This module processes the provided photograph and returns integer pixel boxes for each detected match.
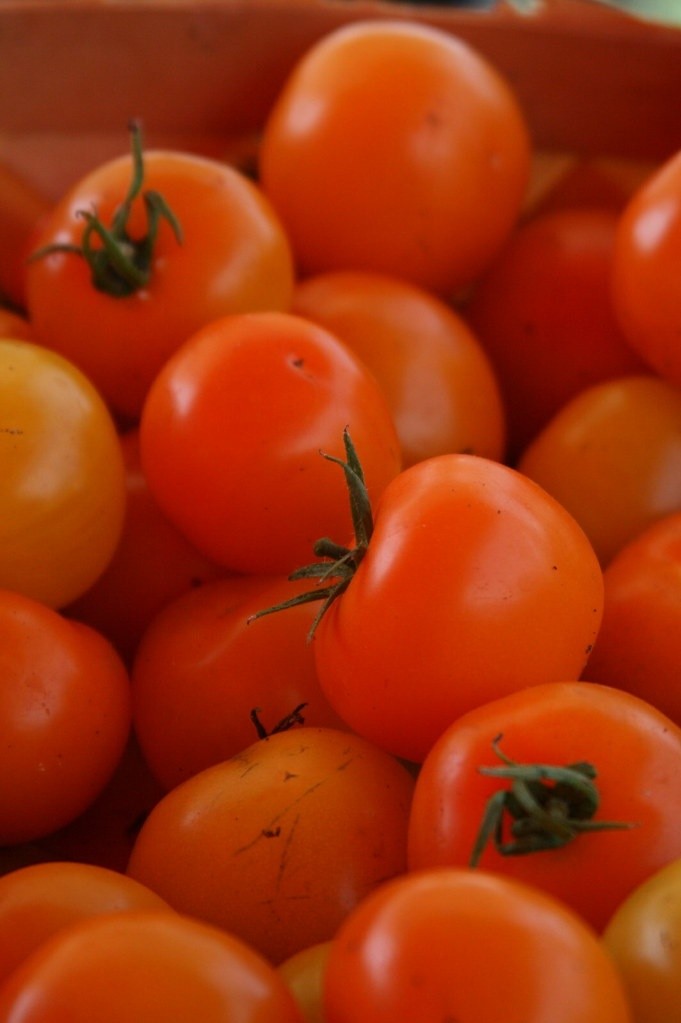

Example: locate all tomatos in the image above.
[0,0,681,1023]
